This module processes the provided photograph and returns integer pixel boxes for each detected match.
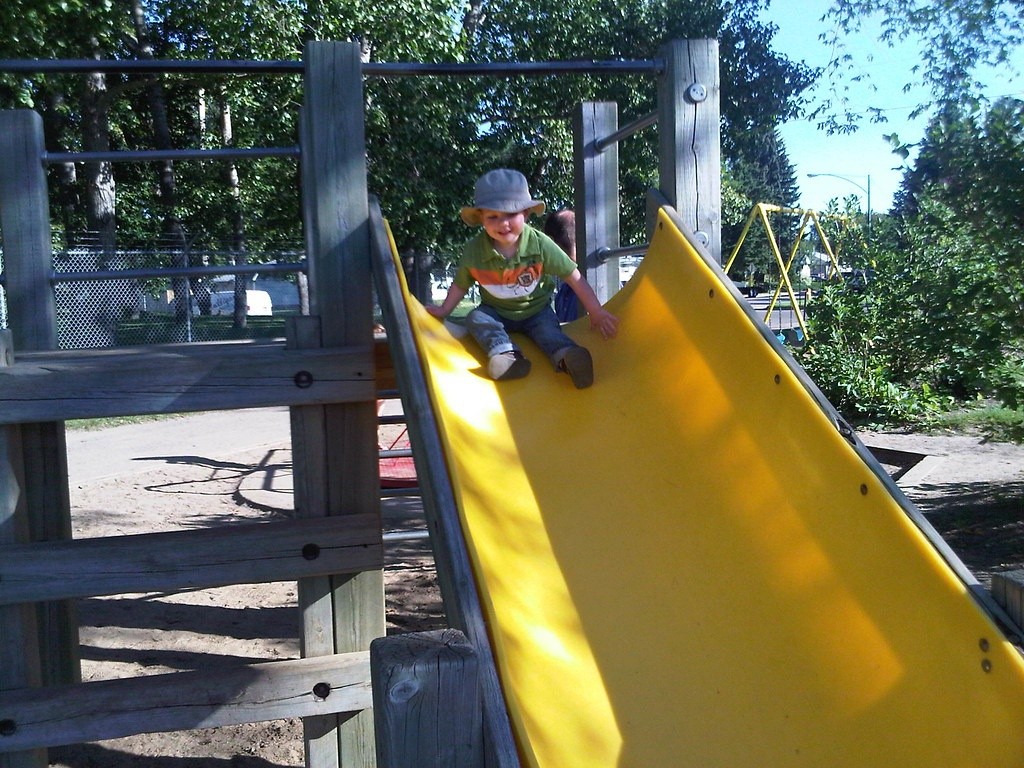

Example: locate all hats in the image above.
[462,169,547,227]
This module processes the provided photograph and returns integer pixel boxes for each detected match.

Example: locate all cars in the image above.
[170,271,299,318]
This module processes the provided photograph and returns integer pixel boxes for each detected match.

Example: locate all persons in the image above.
[546,207,578,324]
[748,267,762,297]
[425,170,617,390]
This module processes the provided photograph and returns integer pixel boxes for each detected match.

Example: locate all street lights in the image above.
[807,173,871,245]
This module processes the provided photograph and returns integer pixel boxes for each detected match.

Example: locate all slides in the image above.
[378,205,1024,767]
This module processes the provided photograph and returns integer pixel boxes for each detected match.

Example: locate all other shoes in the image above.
[489,350,531,381]
[559,346,593,388]
